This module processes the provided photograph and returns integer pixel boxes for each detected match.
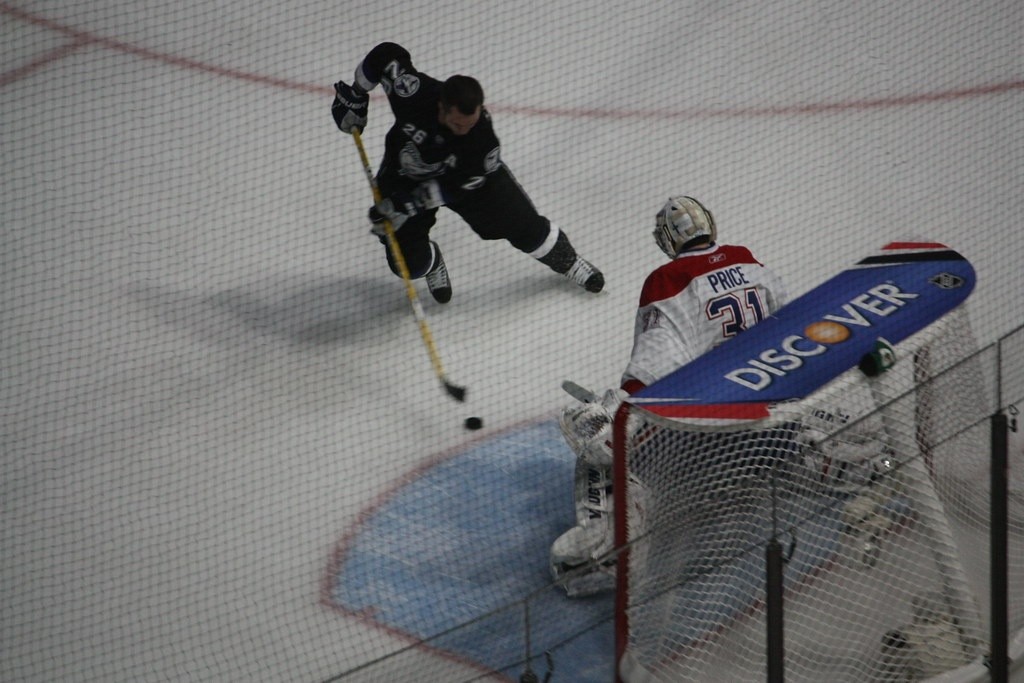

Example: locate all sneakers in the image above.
[562,255,605,293]
[427,242,451,305]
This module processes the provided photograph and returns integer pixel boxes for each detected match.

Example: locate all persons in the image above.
[331,42,606,305]
[548,195,877,599]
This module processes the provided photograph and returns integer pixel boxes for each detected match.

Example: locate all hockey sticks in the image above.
[334,78,471,406]
[560,380,598,402]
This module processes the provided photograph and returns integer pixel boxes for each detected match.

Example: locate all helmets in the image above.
[654,197,718,260]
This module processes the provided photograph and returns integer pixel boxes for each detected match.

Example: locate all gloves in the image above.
[331,81,370,136]
[560,395,618,467]
[369,196,409,239]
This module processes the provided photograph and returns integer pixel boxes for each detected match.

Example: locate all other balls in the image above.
[464,417,481,430]
